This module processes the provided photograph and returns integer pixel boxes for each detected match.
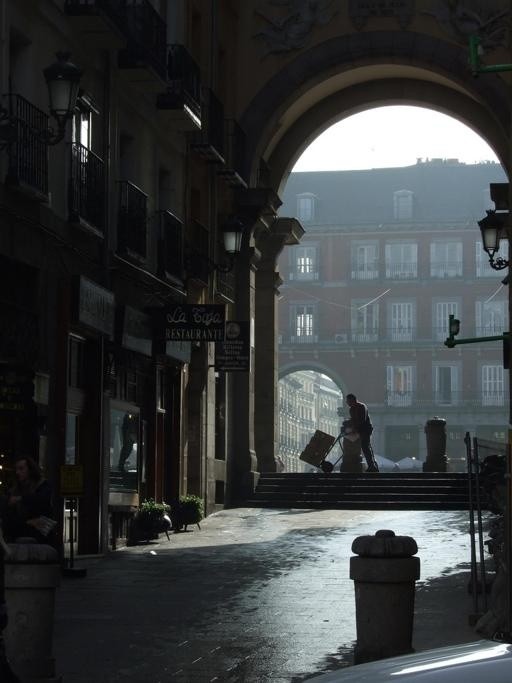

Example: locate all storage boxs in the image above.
[301,429,335,468]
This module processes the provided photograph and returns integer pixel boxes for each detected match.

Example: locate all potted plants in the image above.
[178,493,203,524]
[138,498,173,535]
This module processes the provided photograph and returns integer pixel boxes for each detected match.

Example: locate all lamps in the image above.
[181,210,248,283]
[475,208,510,271]
[208,362,223,378]
[0,45,84,163]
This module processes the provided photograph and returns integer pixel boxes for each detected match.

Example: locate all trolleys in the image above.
[299,419,356,473]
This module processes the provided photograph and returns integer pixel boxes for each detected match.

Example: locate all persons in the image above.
[340,394,382,471]
[116,414,137,475]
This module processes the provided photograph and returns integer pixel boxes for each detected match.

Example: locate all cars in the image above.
[303,637,512,682]
